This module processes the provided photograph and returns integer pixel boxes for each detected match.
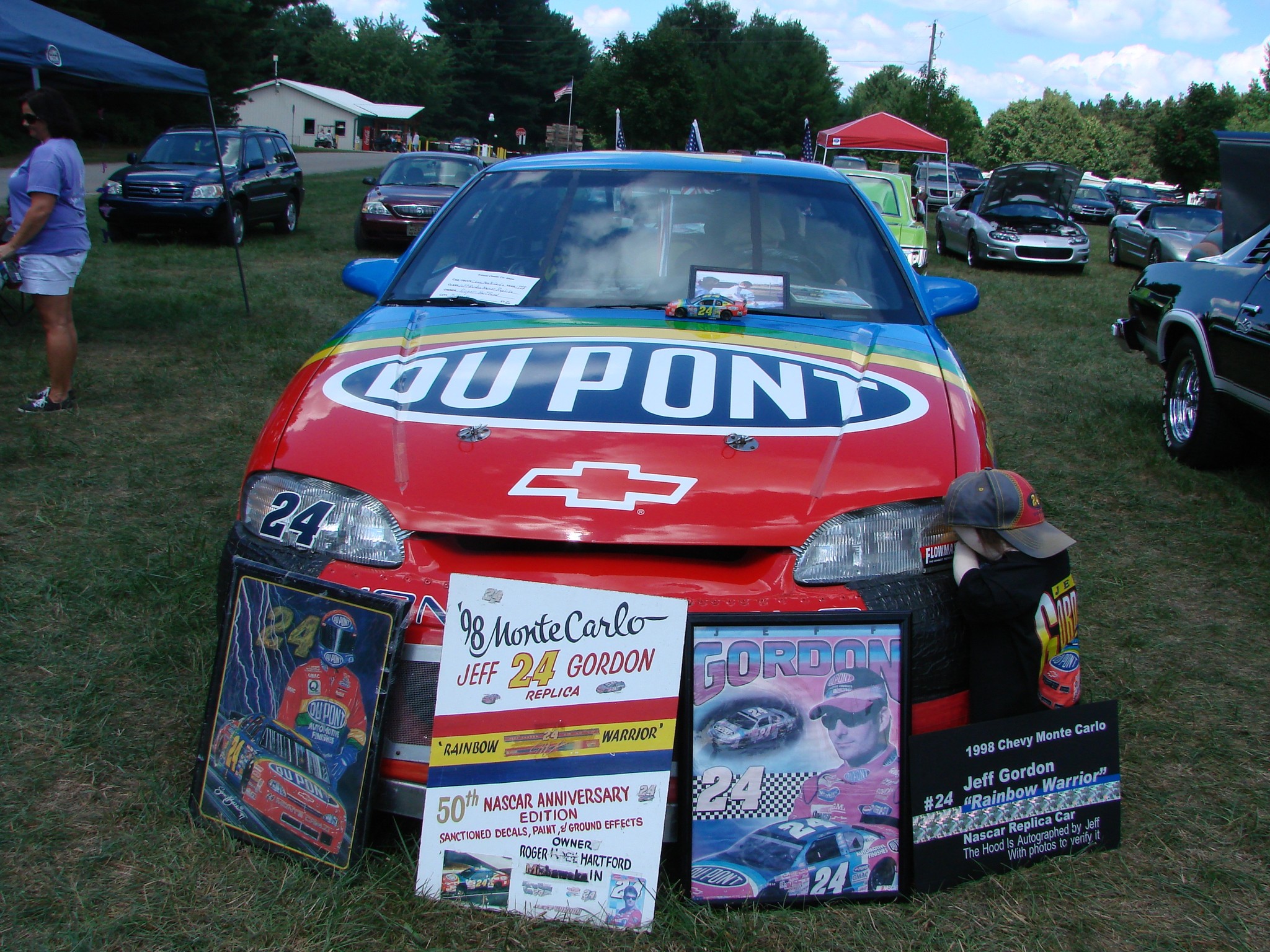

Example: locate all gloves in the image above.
[327,744,358,781]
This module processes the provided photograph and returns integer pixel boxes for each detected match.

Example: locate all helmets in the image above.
[315,609,359,668]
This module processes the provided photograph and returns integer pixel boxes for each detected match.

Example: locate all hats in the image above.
[812,667,887,720]
[944,466,1078,558]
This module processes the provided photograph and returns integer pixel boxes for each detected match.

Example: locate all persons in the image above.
[695,277,759,306]
[943,469,1084,718]
[0,86,89,413]
[609,885,642,930]
[788,666,898,875]
[318,129,420,154]
[276,611,366,793]
[910,186,926,223]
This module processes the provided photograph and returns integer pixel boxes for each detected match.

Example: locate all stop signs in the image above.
[516,128,526,140]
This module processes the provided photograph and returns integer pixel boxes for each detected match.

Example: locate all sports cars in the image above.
[1107,202,1225,271]
[936,160,1090,275]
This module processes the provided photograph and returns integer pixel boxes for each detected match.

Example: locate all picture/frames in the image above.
[687,265,793,314]
[677,611,914,909]
[184,555,411,876]
[789,282,872,310]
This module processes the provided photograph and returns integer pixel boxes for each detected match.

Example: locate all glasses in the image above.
[19,112,39,125]
[821,708,878,729]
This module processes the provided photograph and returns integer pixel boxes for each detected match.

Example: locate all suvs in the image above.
[833,156,867,170]
[753,150,786,160]
[97,124,304,246]
[727,149,750,156]
[1103,180,1162,214]
[910,160,966,210]
[946,161,985,194]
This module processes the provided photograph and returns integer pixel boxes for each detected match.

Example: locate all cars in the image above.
[355,152,493,242]
[1070,184,1116,224]
[1111,131,1270,468]
[207,151,1000,815]
[837,168,927,274]
[449,137,480,156]
[1152,188,1186,205]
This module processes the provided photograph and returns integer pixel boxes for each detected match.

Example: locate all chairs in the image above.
[455,171,471,182]
[1157,213,1182,228]
[405,167,425,182]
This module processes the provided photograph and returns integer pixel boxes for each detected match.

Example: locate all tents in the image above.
[0,0,251,309]
[1212,131,1270,253]
[814,112,949,236]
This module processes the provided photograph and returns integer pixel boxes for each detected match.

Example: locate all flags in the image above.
[680,123,715,194]
[615,118,632,213]
[802,119,813,162]
[554,81,573,102]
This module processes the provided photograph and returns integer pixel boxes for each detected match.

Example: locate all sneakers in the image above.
[27,385,76,401]
[18,395,73,412]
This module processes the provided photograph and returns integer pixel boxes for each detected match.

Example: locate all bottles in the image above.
[0,258,23,289]
